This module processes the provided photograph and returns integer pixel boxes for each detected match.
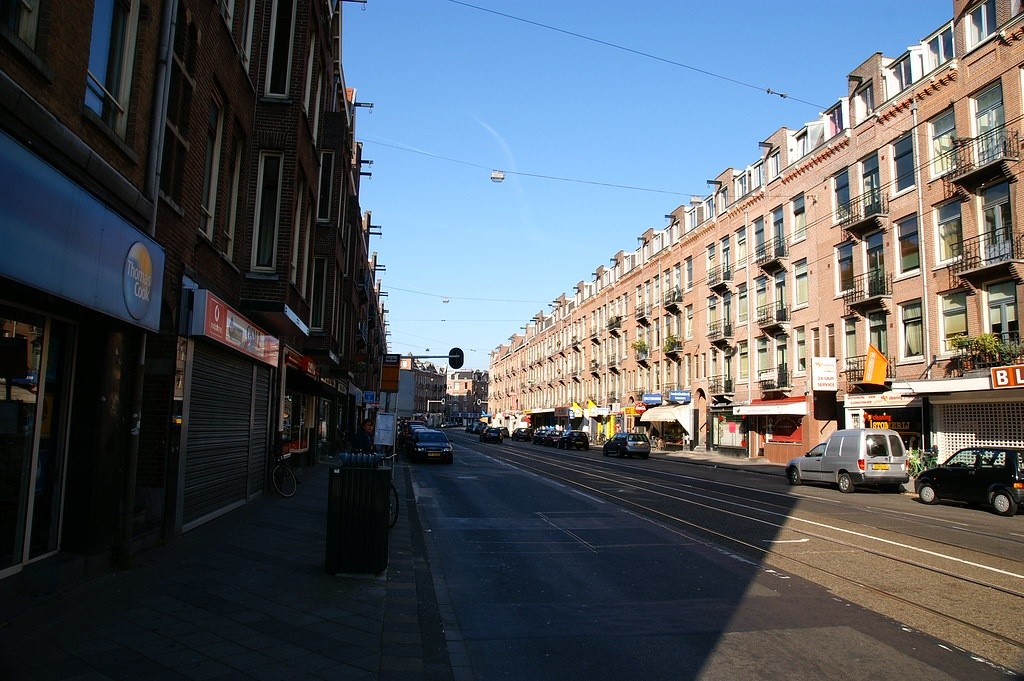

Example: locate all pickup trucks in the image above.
[785,429,909,493]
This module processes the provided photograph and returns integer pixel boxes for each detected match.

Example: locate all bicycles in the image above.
[374,453,399,528]
[274,448,297,497]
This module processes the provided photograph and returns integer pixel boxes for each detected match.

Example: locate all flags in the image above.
[588,400,598,417]
[862,343,887,385]
[573,402,582,418]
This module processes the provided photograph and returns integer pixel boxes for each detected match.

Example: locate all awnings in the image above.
[640,403,678,423]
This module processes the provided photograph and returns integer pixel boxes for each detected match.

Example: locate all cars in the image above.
[542,429,563,448]
[465,422,486,435]
[440,420,458,428]
[532,429,549,445]
[512,428,531,442]
[914,446,1023,516]
[557,430,590,451]
[479,427,503,443]
[410,429,454,464]
[603,432,651,459]
[403,421,429,450]
[497,426,509,438]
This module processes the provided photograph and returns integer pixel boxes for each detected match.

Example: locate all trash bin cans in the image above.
[324,452,392,578]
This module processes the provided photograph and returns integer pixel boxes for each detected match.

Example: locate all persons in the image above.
[351,418,375,455]
[657,437,666,451]
